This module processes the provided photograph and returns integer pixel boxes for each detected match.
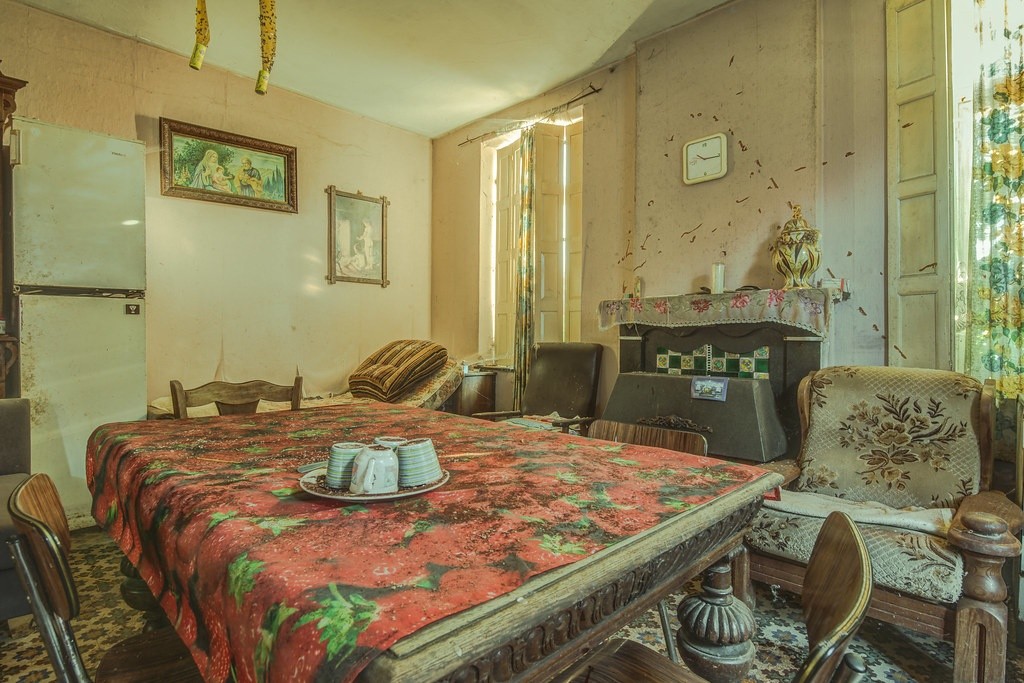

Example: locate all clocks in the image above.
[683,133,728,184]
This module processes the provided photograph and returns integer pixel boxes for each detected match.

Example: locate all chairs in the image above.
[0,398,33,627]
[560,510,871,683]
[734,360,1023,683]
[148,360,464,421]
[471,342,602,435]
[8,471,205,683]
[168,376,306,419]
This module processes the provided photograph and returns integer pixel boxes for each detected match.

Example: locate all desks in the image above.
[84,404,786,682]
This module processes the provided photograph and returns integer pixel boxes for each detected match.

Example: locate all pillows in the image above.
[351,338,447,403]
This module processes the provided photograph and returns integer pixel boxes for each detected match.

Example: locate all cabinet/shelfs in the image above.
[440,371,495,417]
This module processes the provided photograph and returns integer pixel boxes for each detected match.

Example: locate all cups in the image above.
[349,445,399,494]
[374,436,408,457]
[395,438,443,487]
[324,442,366,488]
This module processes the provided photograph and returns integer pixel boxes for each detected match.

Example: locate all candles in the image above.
[711,259,725,295]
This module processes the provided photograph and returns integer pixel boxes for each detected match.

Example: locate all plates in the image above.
[300,468,450,503]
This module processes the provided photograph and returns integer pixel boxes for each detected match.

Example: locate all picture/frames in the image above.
[159,116,299,214]
[324,185,392,288]
